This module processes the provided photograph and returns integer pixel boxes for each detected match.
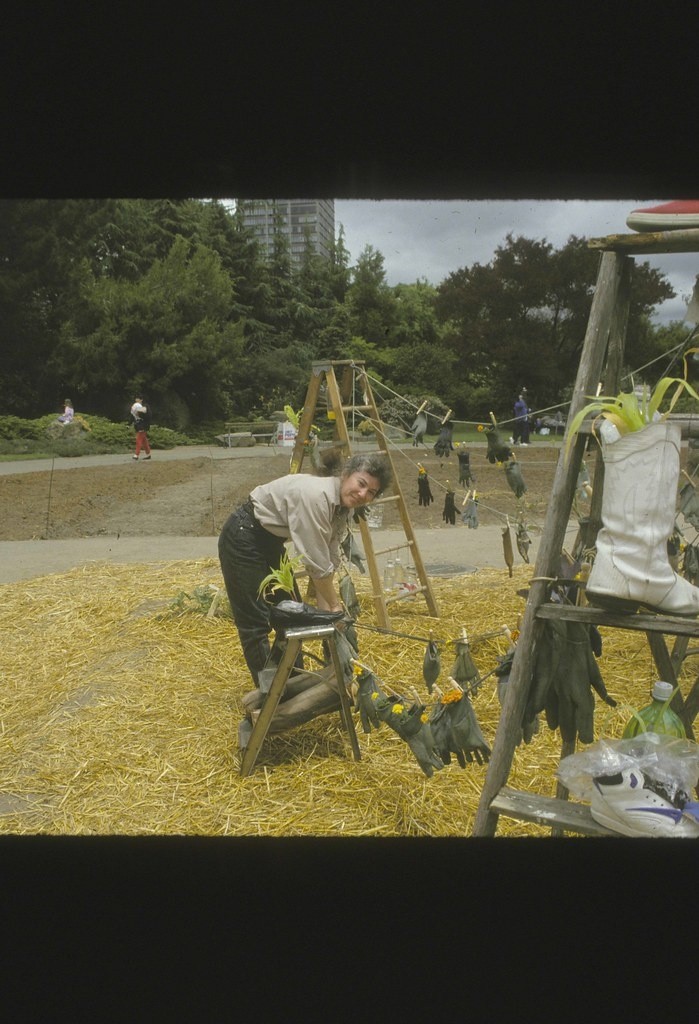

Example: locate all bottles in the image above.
[384,560,418,594]
[326,386,342,420]
[620,682,686,742]
[632,385,663,425]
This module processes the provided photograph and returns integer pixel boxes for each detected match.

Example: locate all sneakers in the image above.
[591,767,699,838]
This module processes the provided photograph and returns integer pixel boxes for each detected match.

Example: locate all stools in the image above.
[240,623,361,777]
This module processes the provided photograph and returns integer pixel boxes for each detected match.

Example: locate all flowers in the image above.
[351,631,519,723]
[418,425,503,499]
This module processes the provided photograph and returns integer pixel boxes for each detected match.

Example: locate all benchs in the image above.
[223,422,277,449]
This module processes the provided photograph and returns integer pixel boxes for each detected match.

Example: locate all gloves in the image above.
[504,461,528,499]
[342,534,366,574]
[410,411,427,446]
[457,452,476,488]
[516,524,532,564]
[461,497,478,529]
[443,492,461,525]
[322,619,617,779]
[353,505,371,523]
[338,575,362,620]
[485,425,513,464]
[417,473,434,507]
[433,421,454,458]
[501,527,514,578]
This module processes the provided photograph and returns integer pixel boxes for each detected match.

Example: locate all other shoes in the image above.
[509,437,531,447]
[132,457,138,460]
[143,455,151,460]
[626,199,699,231]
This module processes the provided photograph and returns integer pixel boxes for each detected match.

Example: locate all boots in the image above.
[584,423,699,615]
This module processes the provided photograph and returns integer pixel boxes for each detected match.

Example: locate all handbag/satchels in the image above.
[58,415,65,422]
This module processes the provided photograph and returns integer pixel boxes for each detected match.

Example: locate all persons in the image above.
[57,399,74,425]
[535,411,566,433]
[217,454,393,688]
[126,394,152,459]
[509,394,531,446]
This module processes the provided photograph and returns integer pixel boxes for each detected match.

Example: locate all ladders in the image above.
[287,354,441,633]
[470,228,699,837]
